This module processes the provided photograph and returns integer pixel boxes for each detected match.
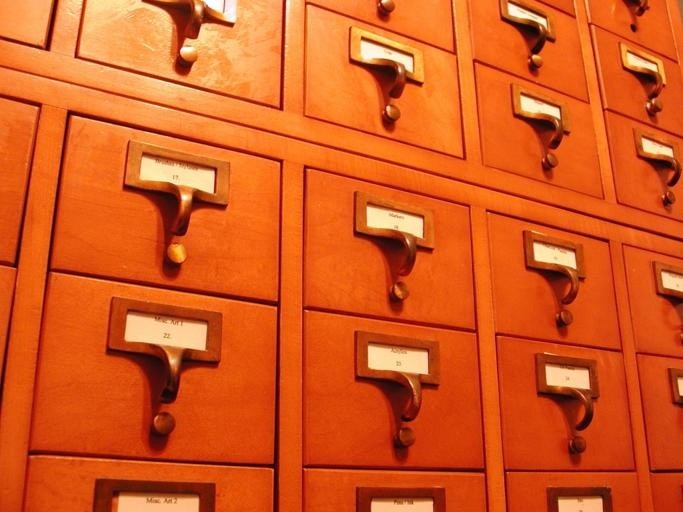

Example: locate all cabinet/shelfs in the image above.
[0,0,683,512]
